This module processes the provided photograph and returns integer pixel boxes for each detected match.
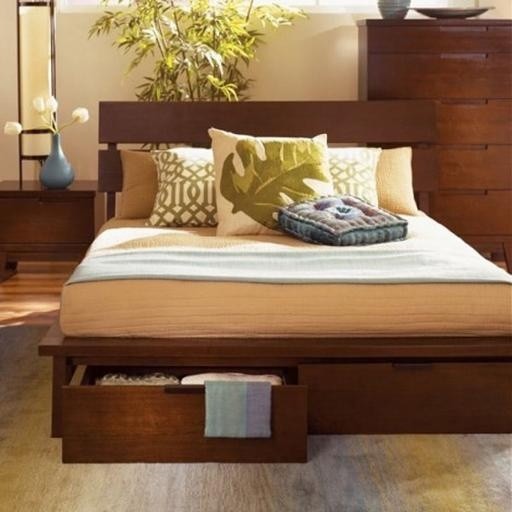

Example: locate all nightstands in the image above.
[0,180,105,285]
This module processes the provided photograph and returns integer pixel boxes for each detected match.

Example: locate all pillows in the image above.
[376,145,419,217]
[144,145,218,229]
[205,128,336,238]
[276,194,409,244]
[118,148,157,221]
[327,147,382,209]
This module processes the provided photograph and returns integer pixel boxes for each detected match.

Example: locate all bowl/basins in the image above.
[377,1,411,21]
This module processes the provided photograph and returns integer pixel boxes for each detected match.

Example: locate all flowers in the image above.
[4,96,89,136]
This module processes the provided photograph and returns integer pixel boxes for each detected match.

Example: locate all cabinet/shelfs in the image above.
[52,357,512,463]
[357,19,512,273]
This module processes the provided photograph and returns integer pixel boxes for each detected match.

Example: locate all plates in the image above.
[412,5,489,20]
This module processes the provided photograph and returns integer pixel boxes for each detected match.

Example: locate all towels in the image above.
[203,381,272,438]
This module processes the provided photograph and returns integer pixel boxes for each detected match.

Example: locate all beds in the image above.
[39,101,512,438]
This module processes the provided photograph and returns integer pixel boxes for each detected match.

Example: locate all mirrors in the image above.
[17,0,56,188]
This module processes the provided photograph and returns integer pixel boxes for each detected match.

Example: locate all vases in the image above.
[39,135,75,188]
